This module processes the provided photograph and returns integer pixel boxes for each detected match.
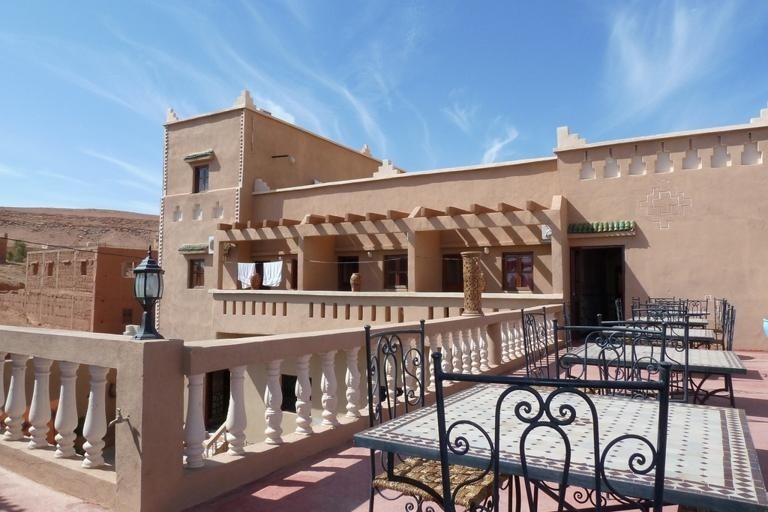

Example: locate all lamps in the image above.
[133,246,165,340]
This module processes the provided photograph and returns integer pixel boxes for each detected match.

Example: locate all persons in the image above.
[241,272,271,290]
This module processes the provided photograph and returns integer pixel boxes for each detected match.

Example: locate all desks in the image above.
[352,384,767,512]
